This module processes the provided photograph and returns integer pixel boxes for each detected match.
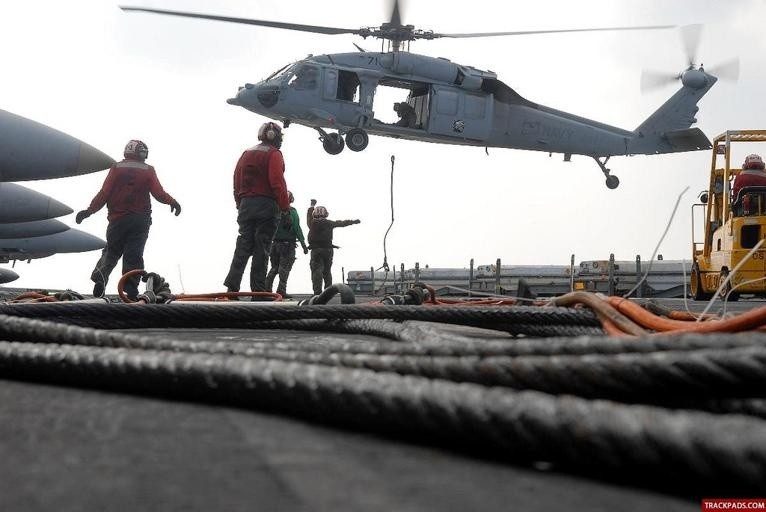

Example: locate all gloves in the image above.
[303,245,308,254]
[170,201,181,216]
[75,210,89,225]
[281,211,293,226]
[352,219,360,224]
[310,199,317,206]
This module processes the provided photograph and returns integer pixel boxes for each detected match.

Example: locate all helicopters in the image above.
[117,0,740,189]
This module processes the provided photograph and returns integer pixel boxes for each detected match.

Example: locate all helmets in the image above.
[746,155,764,169]
[256,121,282,141]
[123,139,149,161]
[287,191,294,204]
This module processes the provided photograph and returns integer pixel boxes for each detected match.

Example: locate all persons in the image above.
[732,153,766,203]
[75,141,181,302]
[306,198,362,295]
[264,189,308,300]
[221,123,295,302]
[390,101,419,130]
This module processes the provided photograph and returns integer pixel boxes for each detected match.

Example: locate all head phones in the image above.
[130,140,149,161]
[287,191,294,202]
[742,154,765,170]
[265,122,276,141]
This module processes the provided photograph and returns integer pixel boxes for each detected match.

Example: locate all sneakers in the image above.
[93,281,106,298]
[225,288,294,304]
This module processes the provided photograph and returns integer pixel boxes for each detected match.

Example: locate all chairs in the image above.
[733,185,766,216]
